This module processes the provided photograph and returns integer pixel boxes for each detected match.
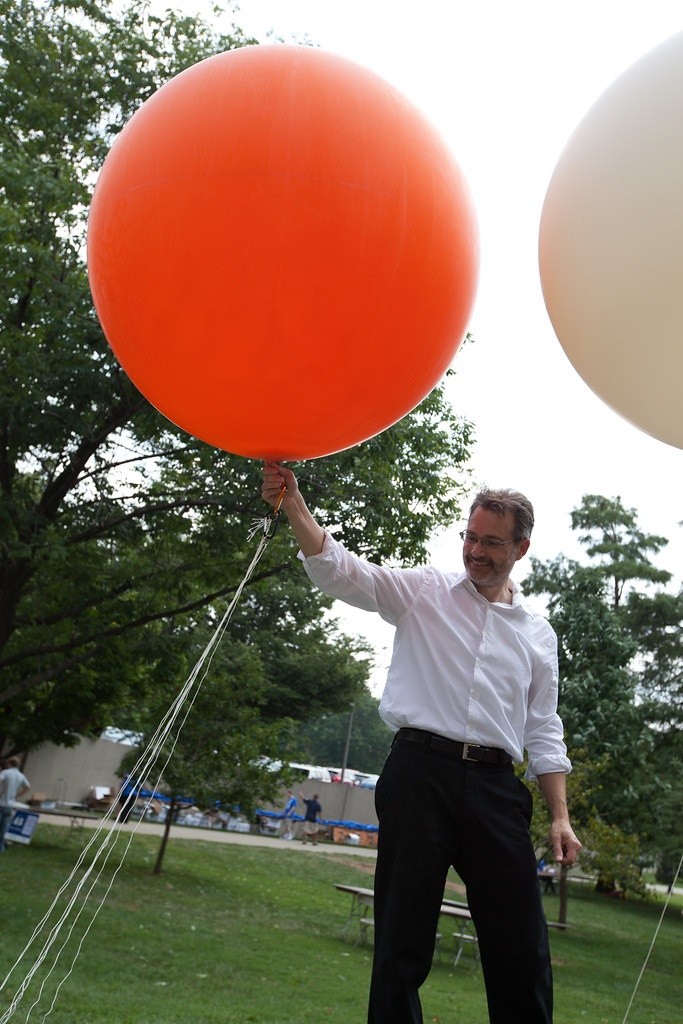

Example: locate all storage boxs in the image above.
[144,801,250,832]
[28,793,57,809]
[345,834,359,844]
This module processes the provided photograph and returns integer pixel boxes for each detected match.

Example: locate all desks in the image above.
[333,882,572,972]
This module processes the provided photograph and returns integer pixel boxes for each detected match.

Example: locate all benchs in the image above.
[353,918,444,965]
[452,931,478,971]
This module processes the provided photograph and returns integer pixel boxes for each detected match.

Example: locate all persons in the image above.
[260,462,584,1023]
[298,790,322,847]
[537,857,558,896]
[282,790,296,840]
[0,756,31,854]
[119,777,140,823]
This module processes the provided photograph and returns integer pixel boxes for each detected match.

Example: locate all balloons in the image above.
[86,45,485,463]
[537,33,683,451]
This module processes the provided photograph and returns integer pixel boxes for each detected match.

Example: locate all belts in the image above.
[393,728,514,772]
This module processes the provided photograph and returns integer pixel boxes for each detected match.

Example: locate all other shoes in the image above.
[284,833,294,840]
[303,840,306,844]
[313,841,318,845]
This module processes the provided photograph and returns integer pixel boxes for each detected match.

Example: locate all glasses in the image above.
[459,529,520,547]
